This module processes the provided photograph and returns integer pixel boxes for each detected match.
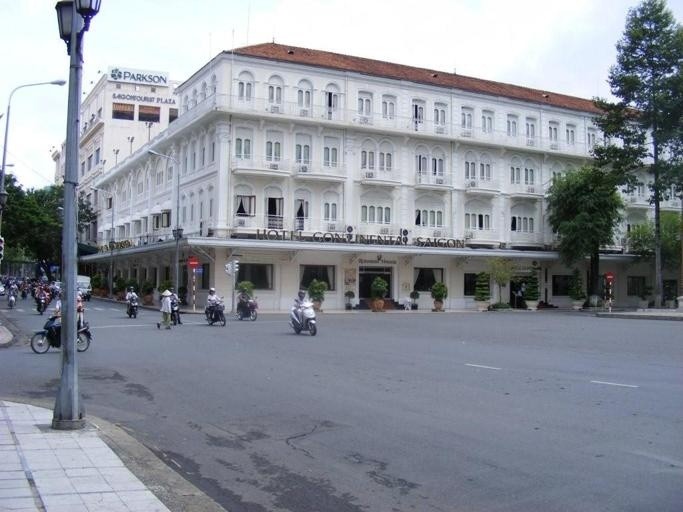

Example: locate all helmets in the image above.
[208,287,215,294]
[129,286,134,292]
[298,291,306,297]
[3,273,58,290]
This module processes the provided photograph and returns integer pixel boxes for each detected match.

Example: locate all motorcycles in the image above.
[170,298,181,326]
[236,296,259,322]
[0,275,84,316]
[288,301,318,337]
[205,296,227,327]
[30,314,93,355]
[126,296,139,319]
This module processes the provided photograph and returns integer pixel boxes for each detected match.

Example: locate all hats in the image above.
[162,289,172,296]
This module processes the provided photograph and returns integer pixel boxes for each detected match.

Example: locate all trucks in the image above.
[76,275,93,302]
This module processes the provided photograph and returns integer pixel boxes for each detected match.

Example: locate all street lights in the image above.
[0,80,67,233]
[89,185,116,299]
[147,149,184,297]
[49,0,103,432]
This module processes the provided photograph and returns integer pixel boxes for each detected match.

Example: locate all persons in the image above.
[126,285,251,330]
[0,276,84,328]
[293,290,314,320]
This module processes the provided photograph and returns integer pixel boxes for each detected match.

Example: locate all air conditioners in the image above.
[346,225,359,242]
[400,228,415,245]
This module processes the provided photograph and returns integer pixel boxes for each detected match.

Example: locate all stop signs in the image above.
[606,272,614,282]
[187,255,199,268]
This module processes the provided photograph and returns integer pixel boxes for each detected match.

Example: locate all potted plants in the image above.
[344,291,355,309]
[571,269,587,310]
[89,275,153,308]
[308,278,329,310]
[473,272,489,312]
[370,276,388,312]
[409,291,420,310]
[523,269,542,310]
[430,281,448,311]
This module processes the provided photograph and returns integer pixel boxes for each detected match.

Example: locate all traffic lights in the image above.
[0,235,4,265]
